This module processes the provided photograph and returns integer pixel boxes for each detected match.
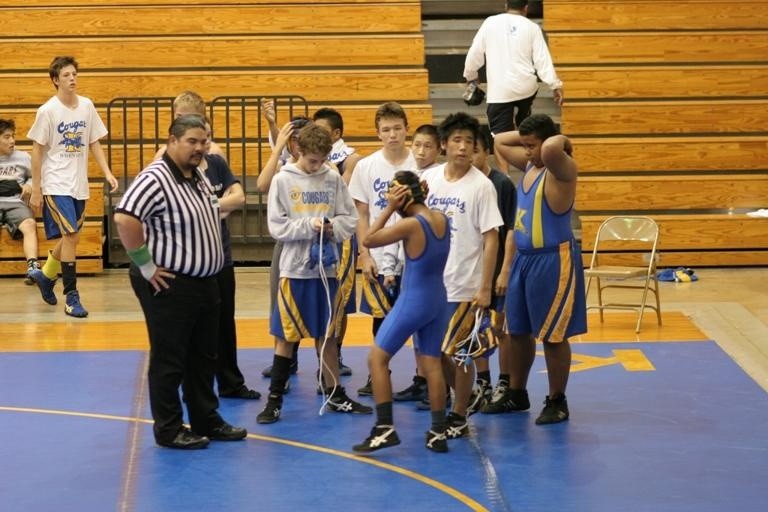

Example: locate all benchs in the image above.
[0,181,105,275]
[582,216,767,266]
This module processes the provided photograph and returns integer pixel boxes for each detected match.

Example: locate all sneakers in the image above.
[471,379,492,401]
[24,261,40,286]
[468,393,485,417]
[352,424,400,452]
[445,416,469,440]
[262,357,299,377]
[336,358,352,376]
[426,430,448,453]
[416,393,451,412]
[481,388,530,414]
[324,387,373,415]
[358,373,372,395]
[257,392,283,424]
[536,396,568,425]
[65,295,88,317]
[392,373,429,401]
[28,270,58,305]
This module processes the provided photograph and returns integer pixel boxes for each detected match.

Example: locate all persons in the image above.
[0,116,44,286]
[481,113,587,425]
[27,56,120,318]
[181,116,262,404]
[417,110,504,440]
[260,98,362,378]
[346,101,415,397]
[112,111,250,450]
[381,123,452,411]
[351,169,453,454]
[254,122,374,424]
[256,113,341,394]
[152,89,227,170]
[462,0,564,181]
[467,123,517,407]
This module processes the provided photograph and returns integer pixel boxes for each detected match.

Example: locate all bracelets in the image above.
[127,243,151,266]
[139,259,158,280]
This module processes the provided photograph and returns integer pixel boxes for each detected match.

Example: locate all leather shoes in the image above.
[154,426,211,449]
[218,385,261,399]
[190,418,247,440]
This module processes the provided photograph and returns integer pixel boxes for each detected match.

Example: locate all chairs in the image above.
[585,216,662,333]
